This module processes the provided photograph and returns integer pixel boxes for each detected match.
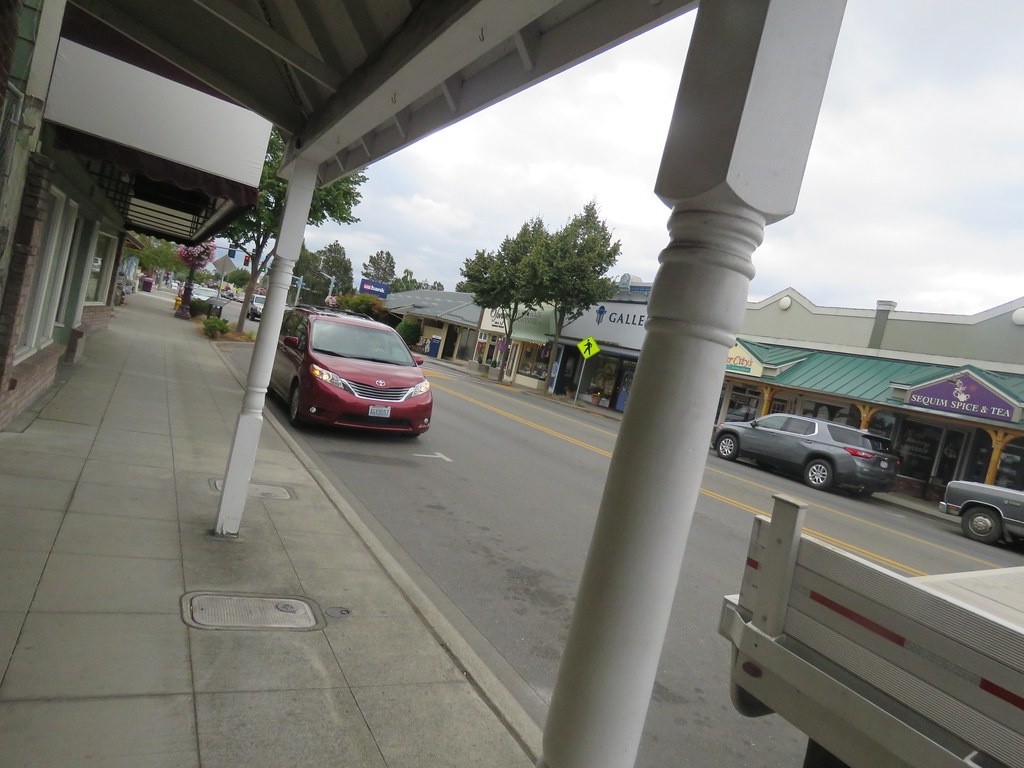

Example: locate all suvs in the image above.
[246,294,266,321]
[268,304,433,439]
[711,413,899,499]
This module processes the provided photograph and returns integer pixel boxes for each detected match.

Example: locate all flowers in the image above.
[178,240,217,268]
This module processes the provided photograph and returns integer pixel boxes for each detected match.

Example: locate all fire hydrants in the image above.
[174,297,181,310]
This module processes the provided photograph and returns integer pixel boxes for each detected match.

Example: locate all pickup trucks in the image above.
[938,480,1024,543]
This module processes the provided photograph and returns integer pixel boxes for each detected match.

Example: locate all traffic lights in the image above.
[244,256,249,266]
[261,262,266,272]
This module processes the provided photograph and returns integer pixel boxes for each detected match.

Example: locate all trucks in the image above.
[718,492,1024,768]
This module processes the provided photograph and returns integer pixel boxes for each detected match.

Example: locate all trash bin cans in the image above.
[142,278,154,293]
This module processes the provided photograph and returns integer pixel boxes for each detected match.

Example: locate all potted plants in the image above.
[588,385,605,405]
